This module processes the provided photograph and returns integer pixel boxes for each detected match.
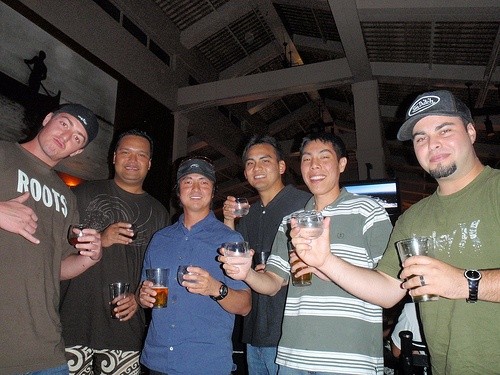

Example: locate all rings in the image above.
[420,275,425,286]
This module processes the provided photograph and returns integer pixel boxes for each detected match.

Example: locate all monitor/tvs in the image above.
[339,179,401,229]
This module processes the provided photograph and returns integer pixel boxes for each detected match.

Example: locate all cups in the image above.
[395,237,440,302]
[109,282,131,319]
[252,244,270,268]
[146,268,169,309]
[119,222,138,241]
[288,241,312,287]
[224,241,248,266]
[67,223,91,246]
[178,265,199,285]
[231,198,249,217]
[295,211,324,239]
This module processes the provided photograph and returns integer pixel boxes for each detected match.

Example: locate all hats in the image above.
[397,90,475,141]
[177,156,216,183]
[54,103,98,148]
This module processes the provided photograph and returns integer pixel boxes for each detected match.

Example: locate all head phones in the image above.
[175,156,219,200]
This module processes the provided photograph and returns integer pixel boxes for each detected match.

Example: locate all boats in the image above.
[0,71,61,113]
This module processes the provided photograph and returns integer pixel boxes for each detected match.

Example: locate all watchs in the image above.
[210,281,228,301]
[464,269,482,303]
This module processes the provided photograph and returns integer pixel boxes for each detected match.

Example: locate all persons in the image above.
[218,132,394,375]
[135,155,252,375]
[0,103,103,375]
[290,90,500,375]
[383,302,433,375]
[223,134,313,375]
[59,130,172,375]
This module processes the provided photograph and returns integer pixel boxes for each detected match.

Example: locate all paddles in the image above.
[23,59,53,99]
